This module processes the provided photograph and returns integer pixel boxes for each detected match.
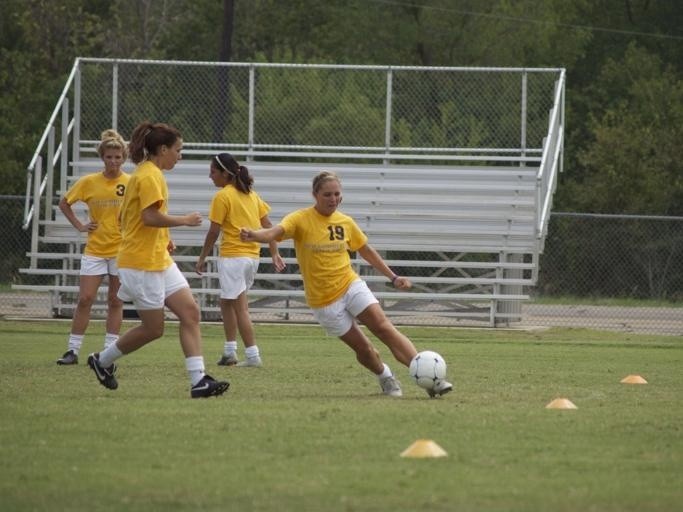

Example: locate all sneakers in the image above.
[217,352,260,366]
[88,349,118,389]
[191,374,230,398]
[378,373,401,396]
[426,380,453,397]
[57,350,78,365]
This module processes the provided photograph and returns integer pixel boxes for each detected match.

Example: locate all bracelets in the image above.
[392,275,398,284]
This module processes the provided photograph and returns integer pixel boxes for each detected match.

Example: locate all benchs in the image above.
[10,154,558,329]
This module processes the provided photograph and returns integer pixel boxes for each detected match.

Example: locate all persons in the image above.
[240,170,453,399]
[195,155,287,368]
[88,119,230,398]
[56,129,129,366]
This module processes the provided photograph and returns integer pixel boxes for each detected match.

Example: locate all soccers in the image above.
[410,350,447,388]
